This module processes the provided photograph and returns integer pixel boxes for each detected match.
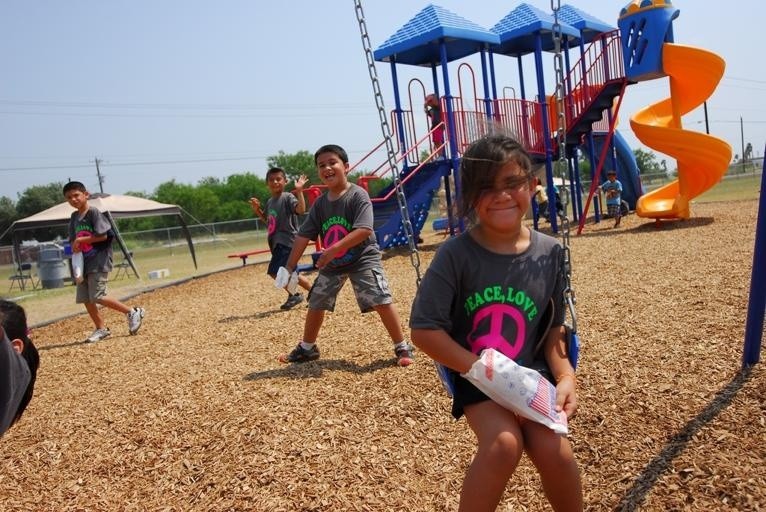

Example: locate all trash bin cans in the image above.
[64,240,71,255]
[38,249,65,289]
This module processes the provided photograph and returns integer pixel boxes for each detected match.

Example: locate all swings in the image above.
[351,0,578,401]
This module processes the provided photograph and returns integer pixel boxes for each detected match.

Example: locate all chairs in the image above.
[112,252,133,281]
[8,264,34,291]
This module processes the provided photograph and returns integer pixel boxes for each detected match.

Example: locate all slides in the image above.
[582,131,647,210]
[630,40,731,220]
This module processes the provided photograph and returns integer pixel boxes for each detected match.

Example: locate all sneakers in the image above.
[127,306,145,335]
[394,343,415,367]
[279,291,304,310]
[86,327,110,344]
[279,342,321,364]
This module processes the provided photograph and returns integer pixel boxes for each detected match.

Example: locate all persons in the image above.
[409,135,583,512]
[534,178,550,222]
[280,145,413,366]
[599,171,623,228]
[1,300,40,434]
[249,168,312,309]
[545,179,568,222]
[424,94,445,161]
[63,182,143,343]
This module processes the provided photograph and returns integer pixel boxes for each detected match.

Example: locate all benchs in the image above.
[226,241,315,266]
